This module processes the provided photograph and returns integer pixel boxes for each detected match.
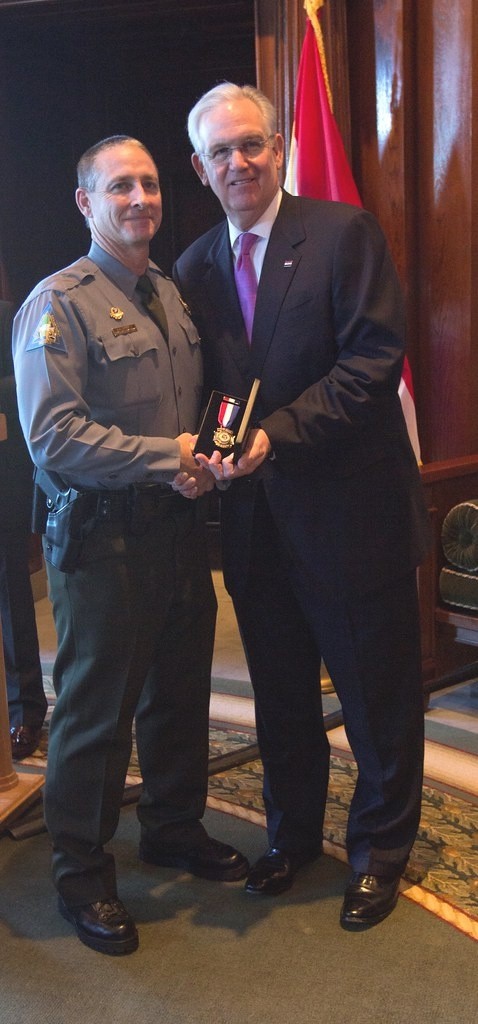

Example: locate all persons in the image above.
[11,134,247,955]
[0,298,49,755]
[169,81,428,929]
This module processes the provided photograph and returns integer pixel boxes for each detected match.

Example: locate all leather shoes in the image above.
[341,871,403,926]
[137,832,246,884]
[55,889,139,957]
[244,846,326,894]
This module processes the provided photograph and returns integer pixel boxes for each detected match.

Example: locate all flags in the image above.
[284,1,422,467]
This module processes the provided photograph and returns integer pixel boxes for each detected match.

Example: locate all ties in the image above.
[136,275,170,341]
[236,233,260,346]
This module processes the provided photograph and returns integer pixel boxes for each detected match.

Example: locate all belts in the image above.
[92,488,191,524]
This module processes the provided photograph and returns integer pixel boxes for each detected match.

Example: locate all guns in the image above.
[34,469,79,510]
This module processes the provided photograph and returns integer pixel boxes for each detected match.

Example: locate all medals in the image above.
[213,426,235,449]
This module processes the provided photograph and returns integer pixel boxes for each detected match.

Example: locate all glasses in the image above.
[200,132,274,166]
